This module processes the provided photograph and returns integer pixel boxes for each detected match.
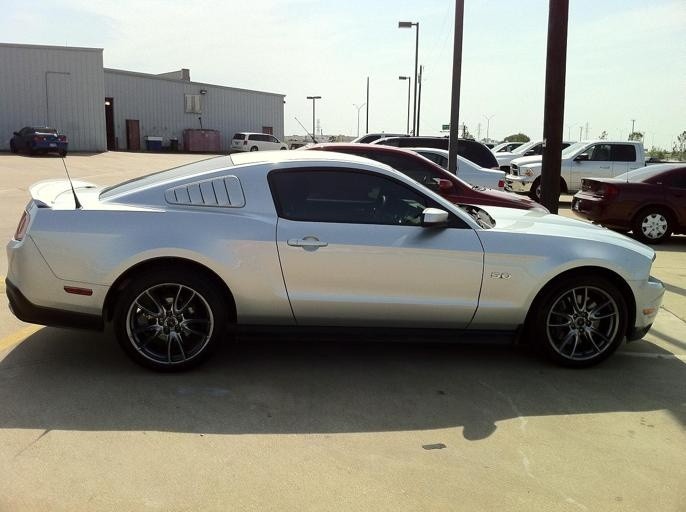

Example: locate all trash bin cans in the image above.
[170,138,179,153]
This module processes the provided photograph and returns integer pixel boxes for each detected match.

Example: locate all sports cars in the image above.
[0,142,671,378]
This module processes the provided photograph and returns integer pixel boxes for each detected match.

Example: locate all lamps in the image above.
[200,90,207,94]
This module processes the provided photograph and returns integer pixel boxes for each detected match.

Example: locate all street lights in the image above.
[351,102,367,137]
[397,21,419,136]
[398,76,410,135]
[306,96,323,139]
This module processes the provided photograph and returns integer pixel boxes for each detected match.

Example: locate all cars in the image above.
[8,125,69,157]
